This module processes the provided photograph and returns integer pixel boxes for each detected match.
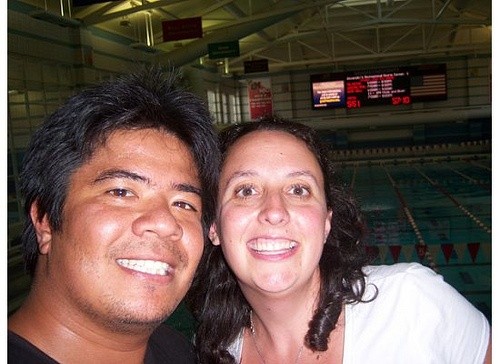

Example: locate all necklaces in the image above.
[246,303,307,364]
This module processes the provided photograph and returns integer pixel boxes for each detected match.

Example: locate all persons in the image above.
[7,62,227,363]
[192,115,491,364]
